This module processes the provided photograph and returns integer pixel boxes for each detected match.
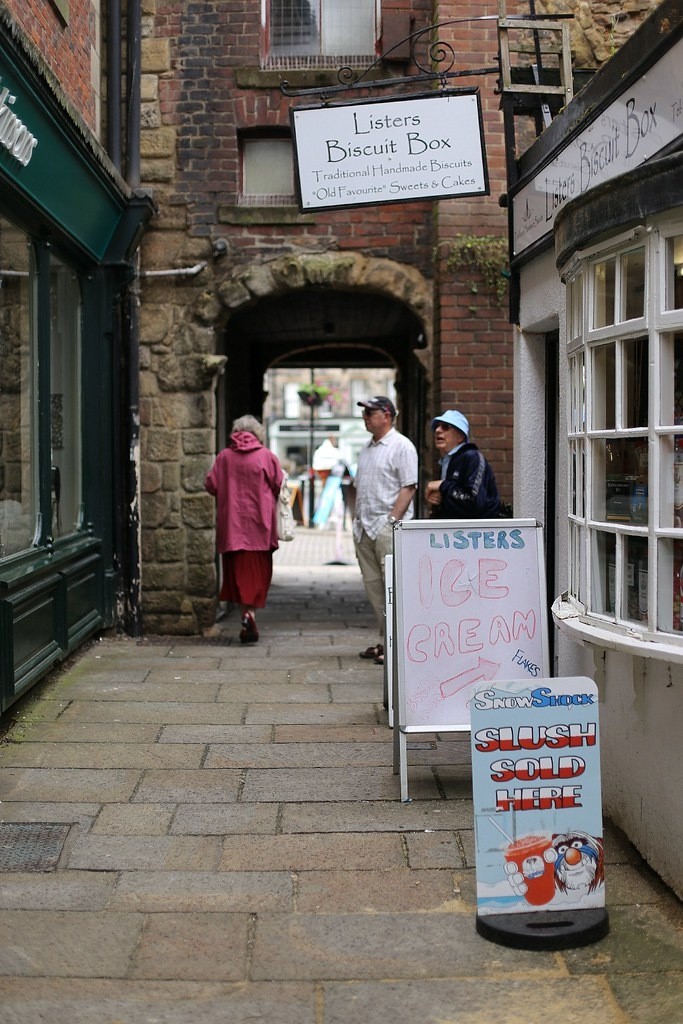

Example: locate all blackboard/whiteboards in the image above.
[383,553,397,726]
[392,518,550,735]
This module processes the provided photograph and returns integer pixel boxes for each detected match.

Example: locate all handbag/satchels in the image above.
[276,468,296,542]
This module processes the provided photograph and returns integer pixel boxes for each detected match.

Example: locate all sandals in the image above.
[359,644,384,658]
[375,655,384,663]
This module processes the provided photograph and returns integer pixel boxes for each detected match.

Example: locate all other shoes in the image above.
[241,611,258,641]
[239,629,248,643]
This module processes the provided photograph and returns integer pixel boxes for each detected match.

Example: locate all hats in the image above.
[431,410,471,443]
[357,396,397,418]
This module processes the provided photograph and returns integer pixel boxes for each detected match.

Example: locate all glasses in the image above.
[361,410,383,417]
[433,423,456,431]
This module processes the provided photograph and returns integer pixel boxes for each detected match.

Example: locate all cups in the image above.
[503,831,556,906]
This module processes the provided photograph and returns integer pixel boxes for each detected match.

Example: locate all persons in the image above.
[350,396,418,666]
[204,415,284,653]
[423,410,500,520]
[313,438,343,485]
